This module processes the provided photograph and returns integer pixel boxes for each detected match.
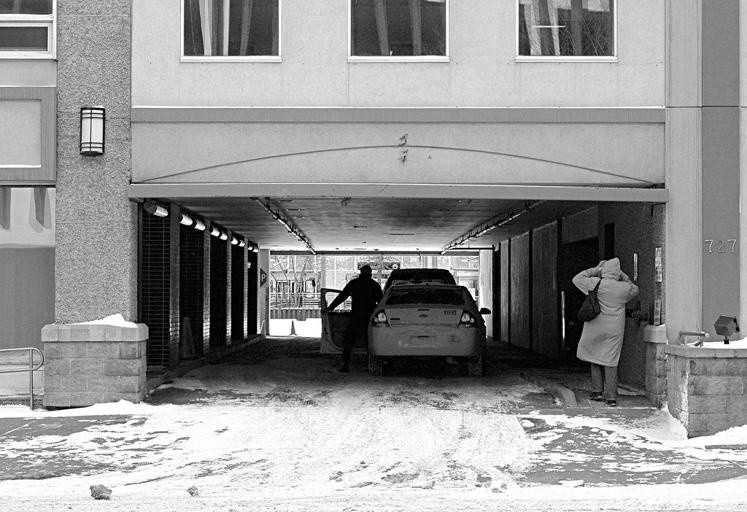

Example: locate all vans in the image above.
[381,267,457,294]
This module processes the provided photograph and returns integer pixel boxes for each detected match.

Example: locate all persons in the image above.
[571,255,641,410]
[319,262,385,374]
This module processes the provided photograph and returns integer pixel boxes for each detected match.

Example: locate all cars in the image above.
[319,281,495,379]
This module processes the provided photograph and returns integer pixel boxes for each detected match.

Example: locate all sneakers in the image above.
[589,392,603,400]
[605,400,617,407]
[338,366,350,373]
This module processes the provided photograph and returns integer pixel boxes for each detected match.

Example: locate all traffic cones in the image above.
[290,320,297,335]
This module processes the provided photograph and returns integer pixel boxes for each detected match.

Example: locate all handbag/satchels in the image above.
[577,291,601,322]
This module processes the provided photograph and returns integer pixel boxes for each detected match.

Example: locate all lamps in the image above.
[714,314,740,344]
[79,105,106,155]
[143,200,257,255]
